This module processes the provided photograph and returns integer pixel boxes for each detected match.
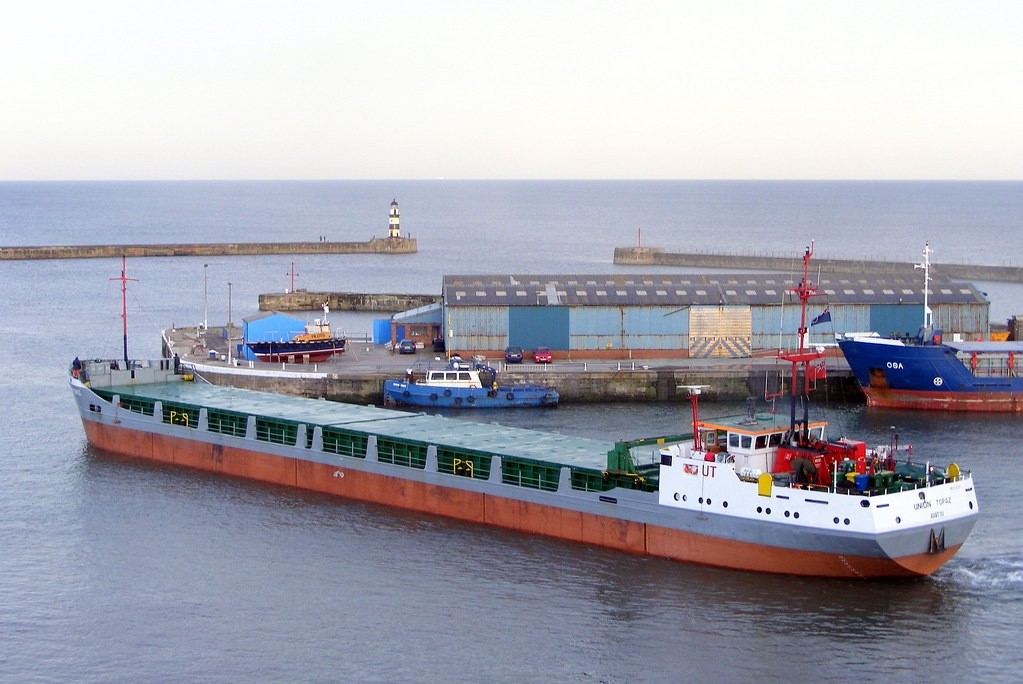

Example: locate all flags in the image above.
[810,306,831,326]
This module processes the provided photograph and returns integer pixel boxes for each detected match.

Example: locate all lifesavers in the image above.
[403,389,514,404]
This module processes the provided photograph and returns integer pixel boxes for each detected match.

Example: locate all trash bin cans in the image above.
[210,352,215,358]
[853,474,869,492]
[873,476,883,488]
[885,474,895,487]
[845,472,860,483]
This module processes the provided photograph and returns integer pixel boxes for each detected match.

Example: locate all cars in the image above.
[399,339,416,354]
[533,347,552,364]
[504,347,524,363]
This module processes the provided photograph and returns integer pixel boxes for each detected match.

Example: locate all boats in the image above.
[68,255,981,583]
[834,243,1023,414]
[245,301,347,363]
[382,346,559,408]
